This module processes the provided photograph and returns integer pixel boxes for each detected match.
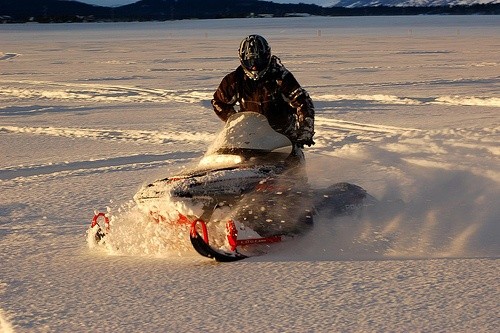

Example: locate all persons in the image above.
[211,34,316,175]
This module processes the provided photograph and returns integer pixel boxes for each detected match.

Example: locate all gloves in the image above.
[296,139,316,148]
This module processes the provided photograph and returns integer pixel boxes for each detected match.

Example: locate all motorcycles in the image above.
[91,112,367,262]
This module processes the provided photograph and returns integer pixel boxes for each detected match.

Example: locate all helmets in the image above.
[238,34,271,81]
